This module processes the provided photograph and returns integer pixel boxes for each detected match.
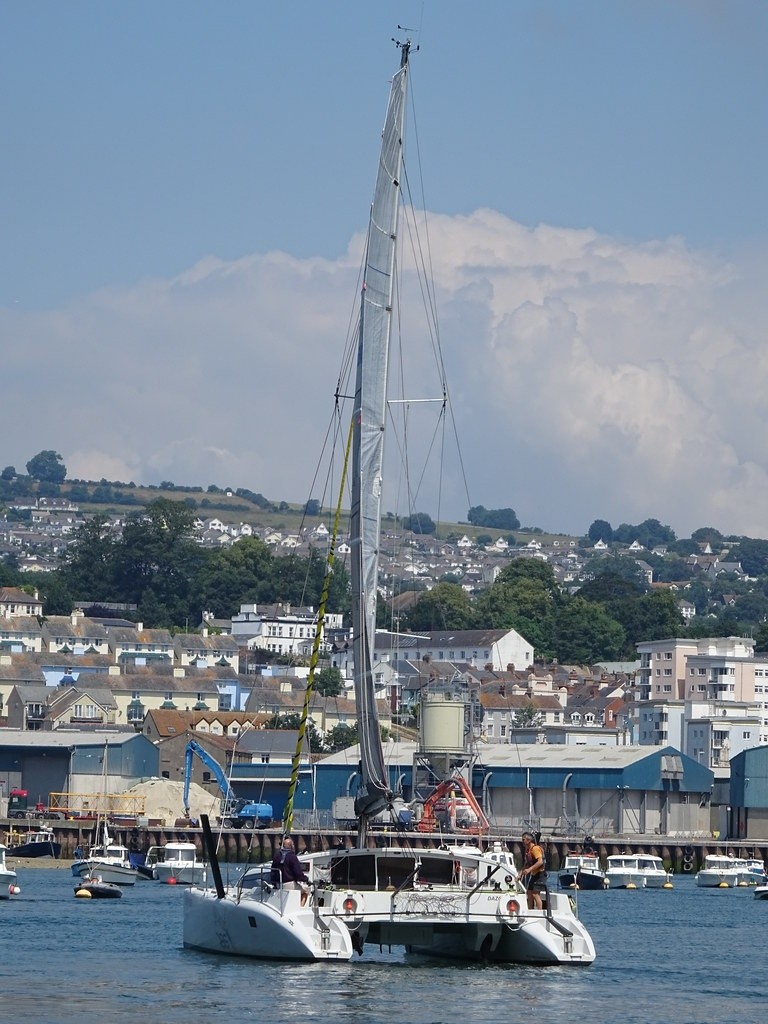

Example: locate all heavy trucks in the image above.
[7,789,59,819]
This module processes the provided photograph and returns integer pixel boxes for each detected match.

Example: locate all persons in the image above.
[269,837,312,908]
[516,832,549,910]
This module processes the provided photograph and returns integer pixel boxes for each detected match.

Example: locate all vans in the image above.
[432,797,478,830]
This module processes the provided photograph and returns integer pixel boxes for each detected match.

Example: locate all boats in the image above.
[71,845,87,876]
[157,843,203,885]
[559,851,605,890]
[134,846,166,880]
[606,854,667,889]
[74,882,122,900]
[695,854,765,888]
[0,844,17,899]
[5,825,61,859]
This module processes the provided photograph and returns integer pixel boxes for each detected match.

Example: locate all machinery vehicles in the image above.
[173,738,274,829]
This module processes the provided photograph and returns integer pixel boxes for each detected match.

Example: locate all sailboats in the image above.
[180,36,597,968]
[79,738,138,884]
